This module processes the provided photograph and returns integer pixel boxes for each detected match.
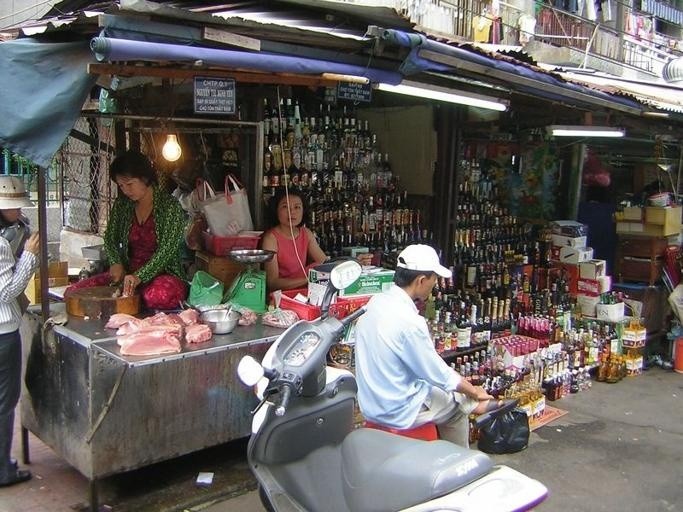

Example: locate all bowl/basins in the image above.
[198,309,242,336]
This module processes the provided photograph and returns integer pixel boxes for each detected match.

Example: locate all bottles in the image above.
[260,88,650,444]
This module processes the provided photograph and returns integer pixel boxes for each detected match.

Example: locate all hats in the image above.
[0,176,35,210]
[394,243,453,280]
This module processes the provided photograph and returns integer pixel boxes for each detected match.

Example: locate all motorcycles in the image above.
[236,260,549,512]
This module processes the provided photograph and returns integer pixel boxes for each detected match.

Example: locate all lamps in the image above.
[162,120,181,162]
[372,79,510,112]
[545,124,625,138]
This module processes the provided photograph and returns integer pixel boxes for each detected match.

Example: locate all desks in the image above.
[439,344,488,366]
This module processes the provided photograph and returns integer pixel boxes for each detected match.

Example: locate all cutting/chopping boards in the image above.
[65,285,143,318]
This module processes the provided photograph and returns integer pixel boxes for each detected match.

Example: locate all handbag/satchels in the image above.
[477,410,529,455]
[198,175,256,236]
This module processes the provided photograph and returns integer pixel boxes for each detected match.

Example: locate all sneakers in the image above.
[1,470,31,488]
[10,458,17,466]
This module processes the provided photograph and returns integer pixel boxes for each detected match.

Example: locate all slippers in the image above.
[472,397,522,430]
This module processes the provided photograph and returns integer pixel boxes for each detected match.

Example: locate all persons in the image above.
[63,150,186,310]
[0,176,40,486]
[258,189,327,292]
[630,181,665,206]
[354,244,520,449]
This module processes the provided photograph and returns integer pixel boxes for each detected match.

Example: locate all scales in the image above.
[222,249,276,313]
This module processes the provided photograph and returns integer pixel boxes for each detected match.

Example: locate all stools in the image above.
[365,422,437,440]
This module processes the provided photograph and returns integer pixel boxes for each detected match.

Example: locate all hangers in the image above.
[525,10,534,17]
[480,8,487,16]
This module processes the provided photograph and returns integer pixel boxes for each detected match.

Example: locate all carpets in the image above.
[529,405,568,433]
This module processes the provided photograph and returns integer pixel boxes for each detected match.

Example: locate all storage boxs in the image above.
[551,206,682,377]
[25,261,68,304]
[520,395,546,423]
[195,251,260,298]
